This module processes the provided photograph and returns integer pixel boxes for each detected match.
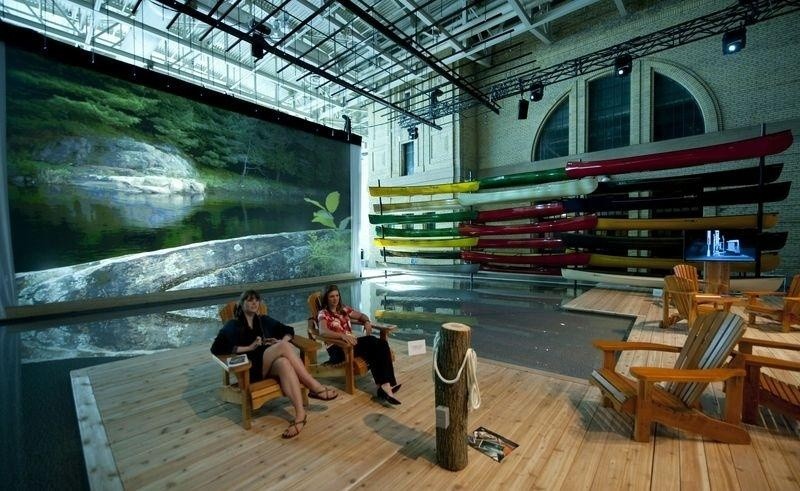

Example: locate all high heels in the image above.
[377,387,401,405]
[391,384,402,394]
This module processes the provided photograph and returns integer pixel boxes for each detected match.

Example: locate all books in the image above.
[226,354,249,367]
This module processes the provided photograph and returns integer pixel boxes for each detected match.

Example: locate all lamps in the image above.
[721,28,747,55]
[531,88,544,102]
[613,55,633,77]
[515,94,529,120]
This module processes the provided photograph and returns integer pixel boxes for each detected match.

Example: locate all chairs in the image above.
[210,298,322,430]
[308,291,396,394]
[673,264,704,294]
[742,274,800,333]
[590,311,752,445]
[724,337,800,425]
[659,275,740,333]
[706,262,732,294]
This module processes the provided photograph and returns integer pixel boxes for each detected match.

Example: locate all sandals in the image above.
[308,387,339,401]
[282,415,307,437]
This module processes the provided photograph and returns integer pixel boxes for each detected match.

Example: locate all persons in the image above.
[318,284,402,406]
[210,289,338,439]
